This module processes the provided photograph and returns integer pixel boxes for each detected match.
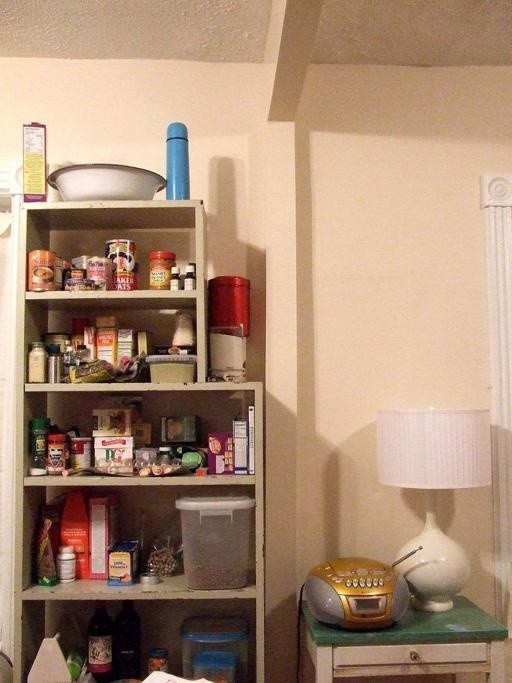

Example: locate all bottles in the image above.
[28,340,90,384]
[169,265,195,291]
[88,600,142,683]
[165,121,190,200]
[29,418,70,476]
[56,545,76,583]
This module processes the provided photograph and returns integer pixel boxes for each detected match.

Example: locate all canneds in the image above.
[148,250,176,290]
[27,239,139,292]
[43,333,70,353]
[48,433,92,475]
[148,648,169,674]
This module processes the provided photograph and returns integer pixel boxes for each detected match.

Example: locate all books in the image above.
[247,404,256,475]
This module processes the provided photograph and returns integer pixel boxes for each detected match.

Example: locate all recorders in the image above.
[304,557,411,631]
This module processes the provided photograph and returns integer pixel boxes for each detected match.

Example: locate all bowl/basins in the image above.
[47,162,168,201]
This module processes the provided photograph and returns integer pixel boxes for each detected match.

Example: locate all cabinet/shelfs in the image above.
[10,203,209,385]
[13,380,267,682]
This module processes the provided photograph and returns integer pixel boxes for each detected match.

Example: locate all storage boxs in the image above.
[147,356,198,384]
[181,614,253,680]
[175,497,254,592]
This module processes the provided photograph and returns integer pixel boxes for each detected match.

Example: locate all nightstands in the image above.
[295,593,510,680]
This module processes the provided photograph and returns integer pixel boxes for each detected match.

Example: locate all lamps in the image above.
[374,409,493,617]
